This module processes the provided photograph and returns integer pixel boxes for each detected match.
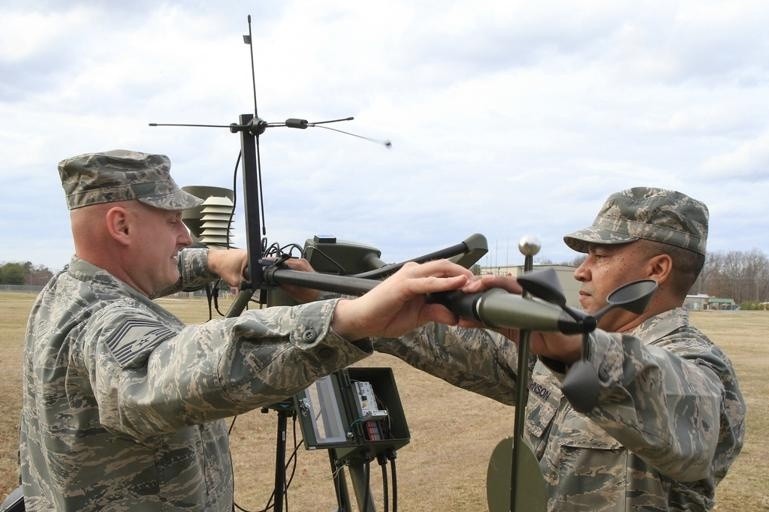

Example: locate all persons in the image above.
[19,147,474,511]
[268,185,747,511]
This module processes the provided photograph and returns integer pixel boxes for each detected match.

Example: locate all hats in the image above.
[563,187,709,256]
[57,150,205,211]
